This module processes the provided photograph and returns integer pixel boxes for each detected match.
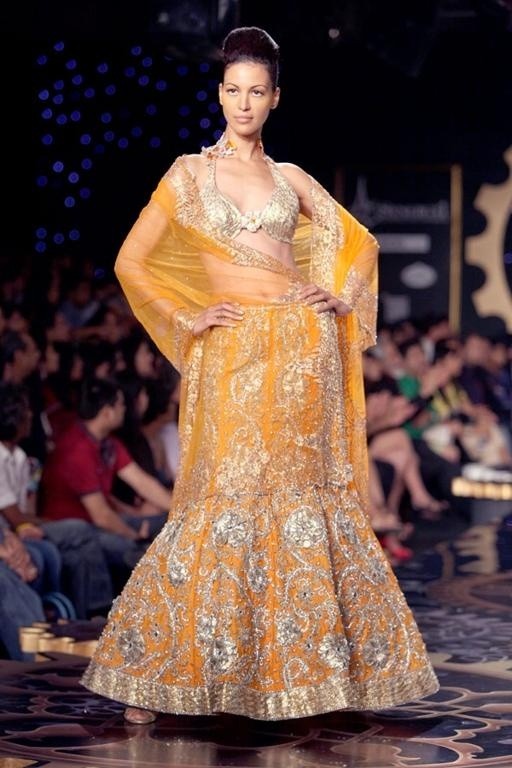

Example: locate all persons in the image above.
[79,24,442,725]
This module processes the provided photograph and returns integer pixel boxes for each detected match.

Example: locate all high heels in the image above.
[123,705,161,724]
